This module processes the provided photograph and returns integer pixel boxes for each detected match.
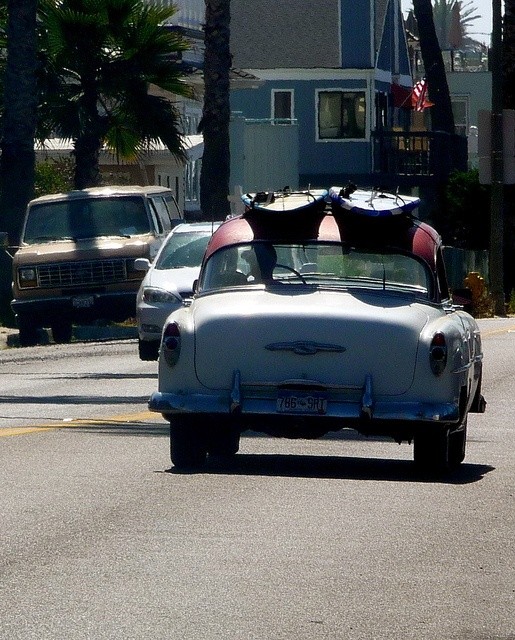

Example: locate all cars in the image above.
[132,221,224,360]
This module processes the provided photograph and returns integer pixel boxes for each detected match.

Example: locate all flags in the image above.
[412,76,427,113]
[391,85,433,111]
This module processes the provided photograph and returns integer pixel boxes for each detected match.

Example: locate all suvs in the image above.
[149,186,485,473]
[2,185,181,342]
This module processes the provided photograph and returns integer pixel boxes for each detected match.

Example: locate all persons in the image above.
[247,244,283,285]
